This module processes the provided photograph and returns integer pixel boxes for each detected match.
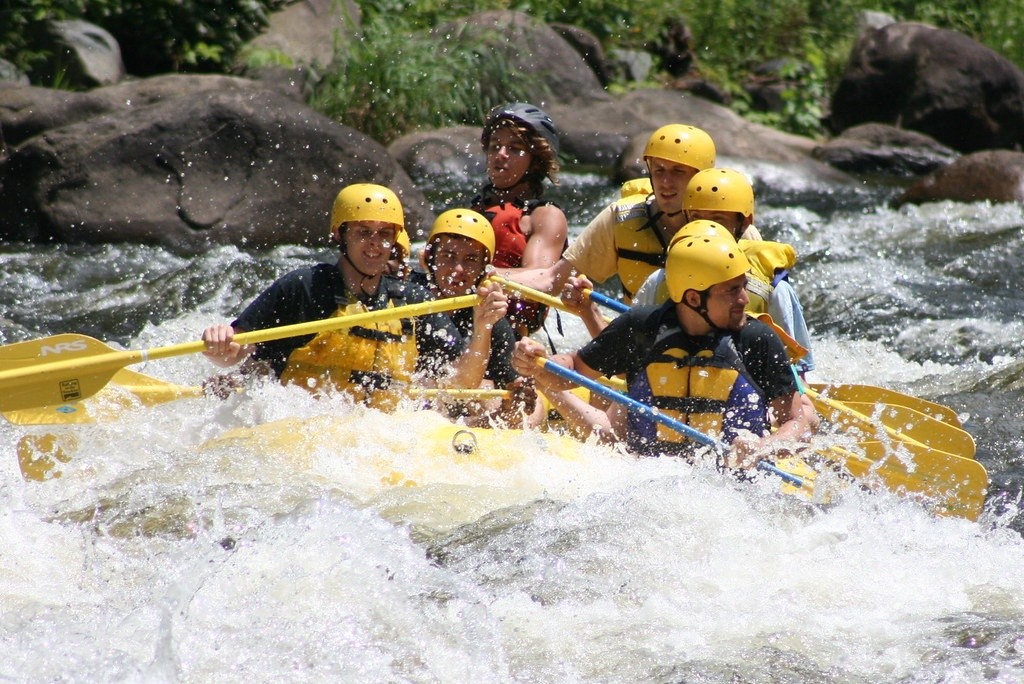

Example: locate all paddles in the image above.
[819,436,988,523]
[577,279,977,459]
[533,355,836,506]
[0,292,482,413]
[119,364,511,408]
[498,274,963,429]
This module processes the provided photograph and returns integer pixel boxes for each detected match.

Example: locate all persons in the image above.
[559,168,816,382]
[537,219,821,447]
[471,102,568,338]
[512,234,811,482]
[501,124,763,307]
[382,226,502,417]
[408,208,545,430]
[201,183,507,413]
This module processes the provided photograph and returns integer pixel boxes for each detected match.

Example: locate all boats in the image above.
[188,372,830,555]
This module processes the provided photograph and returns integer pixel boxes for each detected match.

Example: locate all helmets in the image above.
[682,167,755,224]
[667,219,737,255]
[666,237,751,303]
[642,123,715,170]
[393,225,410,258]
[490,103,559,160]
[329,183,404,245]
[424,208,496,269]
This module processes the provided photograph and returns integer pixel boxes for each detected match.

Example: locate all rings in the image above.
[566,292,571,299]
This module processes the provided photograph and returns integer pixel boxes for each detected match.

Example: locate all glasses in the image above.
[390,246,402,261]
